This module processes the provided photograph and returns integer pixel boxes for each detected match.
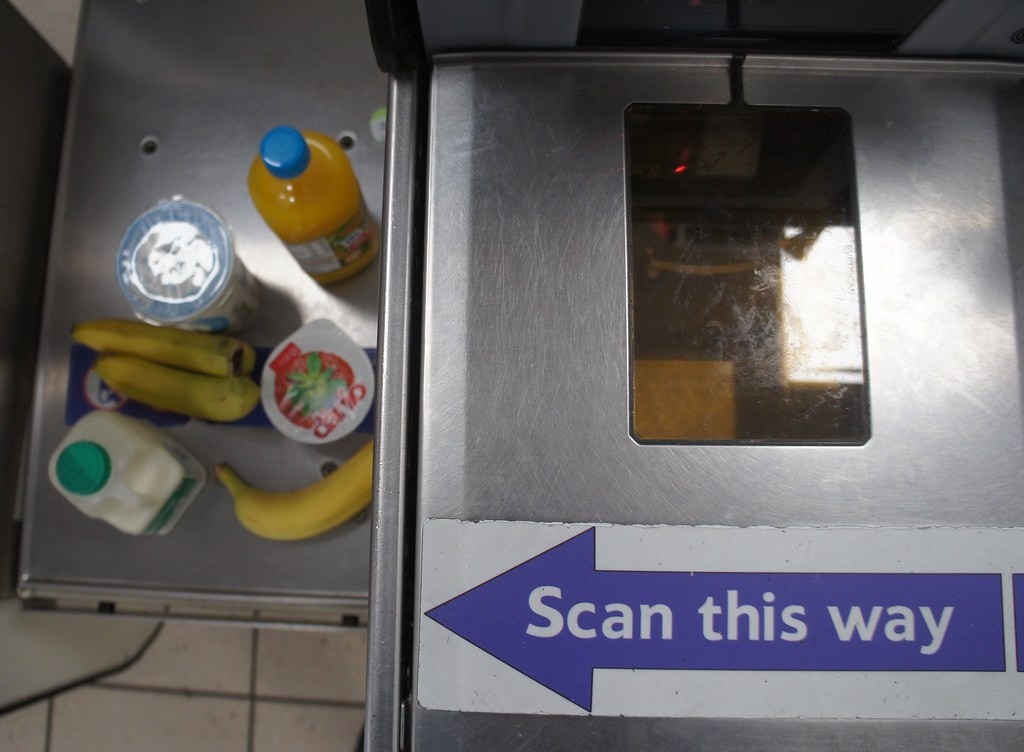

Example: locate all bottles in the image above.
[48,408,206,540]
[247,123,381,287]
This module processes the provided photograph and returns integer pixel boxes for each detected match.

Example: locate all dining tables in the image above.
[15,0,391,604]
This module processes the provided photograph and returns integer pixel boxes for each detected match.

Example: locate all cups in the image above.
[114,200,262,335]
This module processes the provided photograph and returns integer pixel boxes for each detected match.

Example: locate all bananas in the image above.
[213,439,374,540]
[95,357,258,421]
[69,322,253,375]
